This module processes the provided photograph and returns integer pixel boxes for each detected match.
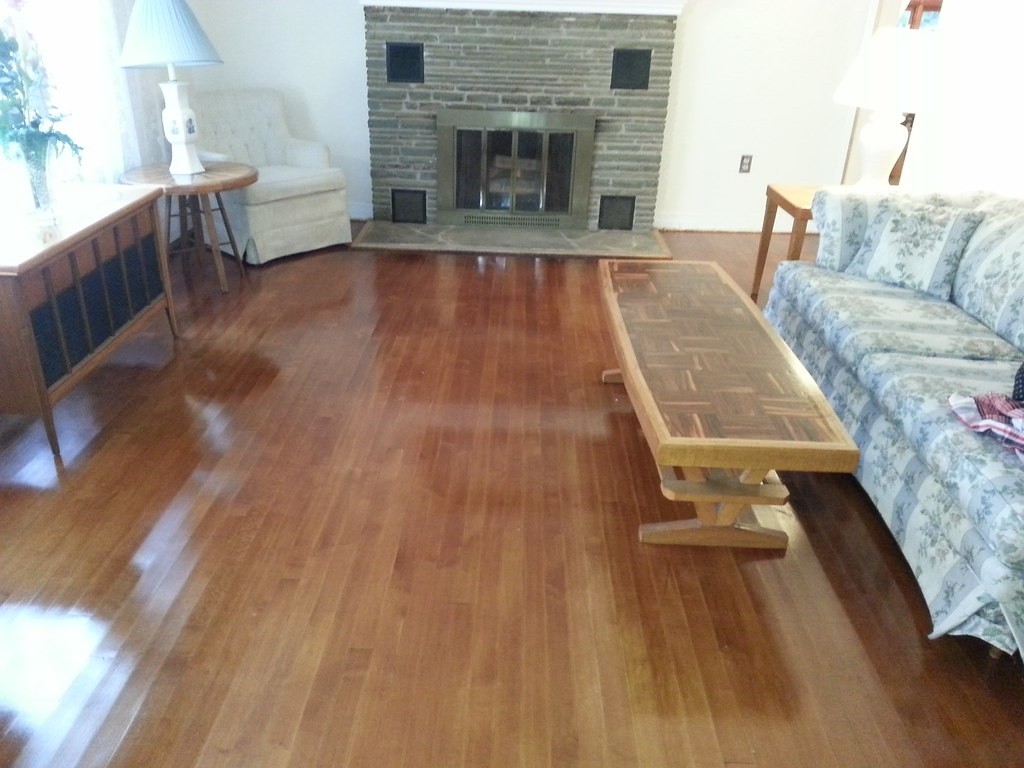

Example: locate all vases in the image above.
[21,134,57,212]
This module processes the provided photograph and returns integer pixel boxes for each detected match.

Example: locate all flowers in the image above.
[0,27,83,163]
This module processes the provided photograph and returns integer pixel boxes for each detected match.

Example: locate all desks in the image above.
[0,177,181,487]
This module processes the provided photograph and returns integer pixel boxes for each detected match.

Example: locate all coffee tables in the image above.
[590,259,858,549]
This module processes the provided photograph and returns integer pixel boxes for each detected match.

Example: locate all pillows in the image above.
[844,186,987,301]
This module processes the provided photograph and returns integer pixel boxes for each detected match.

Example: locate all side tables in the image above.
[121,162,259,294]
[750,186,819,311]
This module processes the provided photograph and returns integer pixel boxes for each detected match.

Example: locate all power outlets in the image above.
[739,154,753,173]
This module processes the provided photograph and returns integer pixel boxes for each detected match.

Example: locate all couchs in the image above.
[762,184,1024,667]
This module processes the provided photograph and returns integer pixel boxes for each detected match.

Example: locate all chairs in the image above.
[199,84,353,266]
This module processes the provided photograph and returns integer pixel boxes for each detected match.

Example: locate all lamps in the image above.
[117,3,226,177]
